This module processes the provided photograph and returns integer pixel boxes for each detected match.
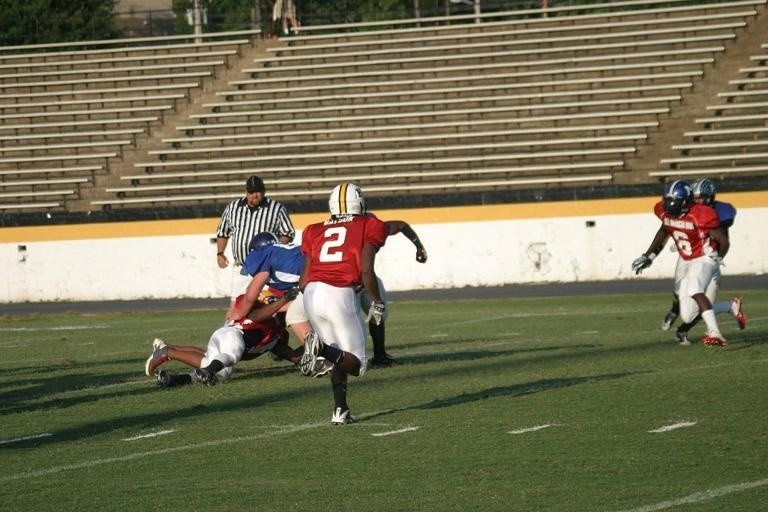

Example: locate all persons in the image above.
[632,179,746,351]
[144,330,303,375]
[225,231,335,378]
[636,177,737,345]
[354,220,427,367]
[300,182,384,426]
[283,0,298,36]
[216,175,295,309]
[157,285,299,387]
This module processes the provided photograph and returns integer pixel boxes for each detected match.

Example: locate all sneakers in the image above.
[662,298,746,347]
[144,336,397,425]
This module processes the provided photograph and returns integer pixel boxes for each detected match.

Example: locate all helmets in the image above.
[663,180,693,216]
[692,178,717,204]
[328,183,366,216]
[249,232,277,252]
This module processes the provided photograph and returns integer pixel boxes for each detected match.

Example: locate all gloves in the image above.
[416,244,427,263]
[365,303,386,327]
[632,253,654,275]
[710,256,725,267]
[284,286,299,302]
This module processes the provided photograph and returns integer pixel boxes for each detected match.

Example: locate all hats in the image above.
[247,175,263,192]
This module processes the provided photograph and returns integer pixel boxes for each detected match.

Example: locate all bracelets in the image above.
[218,252,223,255]
